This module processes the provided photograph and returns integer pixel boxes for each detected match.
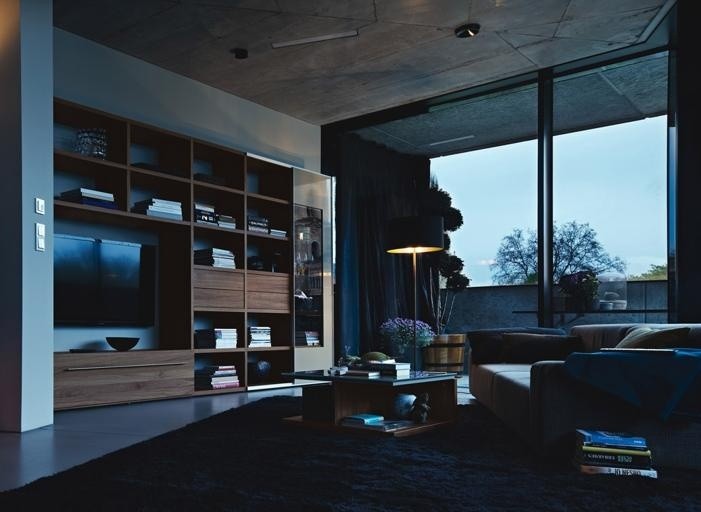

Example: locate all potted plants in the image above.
[415,189,468,371]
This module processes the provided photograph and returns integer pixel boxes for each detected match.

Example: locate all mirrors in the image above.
[293,203,324,348]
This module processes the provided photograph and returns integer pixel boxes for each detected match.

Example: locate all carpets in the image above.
[0,395,700,512]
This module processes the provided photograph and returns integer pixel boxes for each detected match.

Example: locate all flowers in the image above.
[378,317,437,353]
[559,271,600,313]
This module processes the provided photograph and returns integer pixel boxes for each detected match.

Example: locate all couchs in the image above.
[467,322,701,469]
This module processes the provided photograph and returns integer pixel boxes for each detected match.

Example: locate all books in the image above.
[295,330,321,347]
[61,187,117,209]
[194,247,236,270]
[346,361,411,377]
[129,198,183,221]
[576,429,657,480]
[343,413,414,433]
[194,202,237,229]
[247,215,287,238]
[195,326,271,390]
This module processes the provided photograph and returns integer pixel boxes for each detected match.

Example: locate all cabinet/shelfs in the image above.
[53,95,296,412]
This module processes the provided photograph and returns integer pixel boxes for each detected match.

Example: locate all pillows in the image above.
[494,331,580,363]
[469,333,508,364]
[616,326,691,348]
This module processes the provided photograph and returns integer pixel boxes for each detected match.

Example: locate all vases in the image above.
[403,347,424,371]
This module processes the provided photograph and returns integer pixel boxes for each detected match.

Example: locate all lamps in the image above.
[386,215,445,371]
